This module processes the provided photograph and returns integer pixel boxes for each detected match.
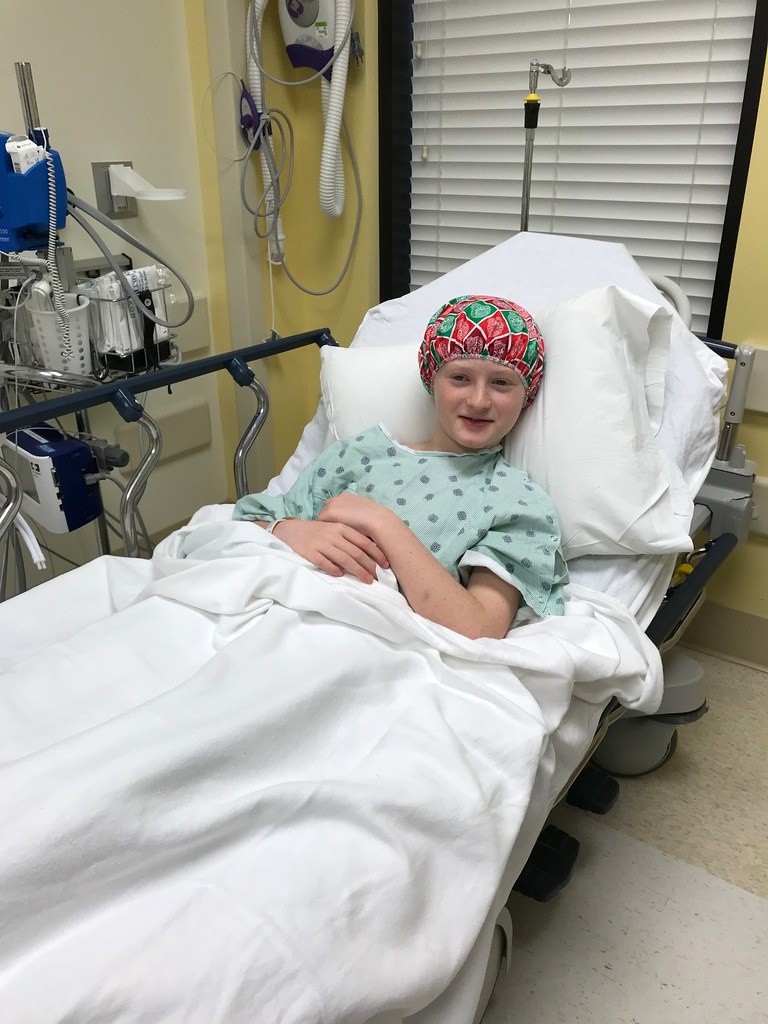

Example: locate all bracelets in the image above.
[265,518,285,536]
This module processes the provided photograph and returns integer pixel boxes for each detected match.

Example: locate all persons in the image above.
[232,292,569,640]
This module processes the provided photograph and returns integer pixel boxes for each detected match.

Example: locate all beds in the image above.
[0,234,755,1024]
